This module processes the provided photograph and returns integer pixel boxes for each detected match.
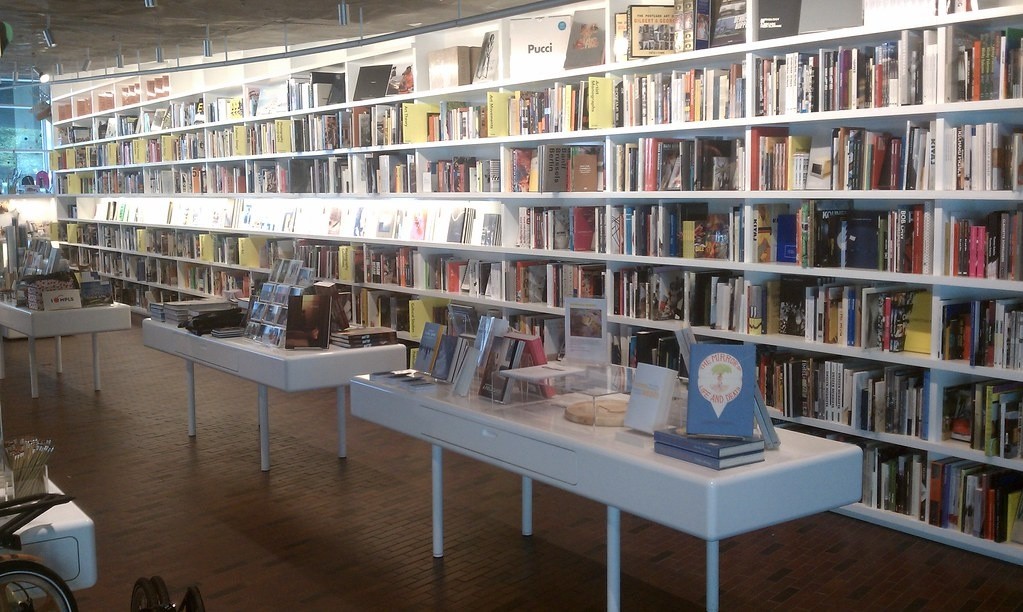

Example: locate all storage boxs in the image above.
[429,46,482,88]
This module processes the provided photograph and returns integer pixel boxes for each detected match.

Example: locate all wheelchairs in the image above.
[0,493,79,612]
[130,576,205,612]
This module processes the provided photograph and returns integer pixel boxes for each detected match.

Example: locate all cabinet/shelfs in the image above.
[45,0,1023,565]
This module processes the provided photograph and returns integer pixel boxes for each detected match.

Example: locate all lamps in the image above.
[33,67,49,83]
[144,0,154,8]
[116,55,124,68]
[81,59,91,71]
[13,72,18,80]
[57,64,63,75]
[337,0,350,25]
[43,29,57,47]
[156,47,164,63]
[203,40,212,57]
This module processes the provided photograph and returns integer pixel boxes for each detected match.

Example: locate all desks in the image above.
[0,300,131,399]
[143,317,407,471]
[350,361,863,612]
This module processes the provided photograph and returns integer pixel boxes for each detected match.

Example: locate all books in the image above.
[119,131,208,194]
[289,103,415,193]
[91,197,502,246]
[351,244,607,360]
[506,80,606,193]
[606,205,1022,548]
[289,65,412,112]
[11,222,98,300]
[751,28,1023,192]
[420,97,501,192]
[98,106,174,139]
[48,142,118,194]
[614,0,981,62]
[149,230,249,326]
[609,59,746,190]
[58,125,93,146]
[98,224,176,310]
[207,119,292,194]
[171,80,289,128]
[412,305,780,472]
[253,234,352,326]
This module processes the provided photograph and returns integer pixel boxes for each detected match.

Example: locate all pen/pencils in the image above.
[4,437,54,499]
